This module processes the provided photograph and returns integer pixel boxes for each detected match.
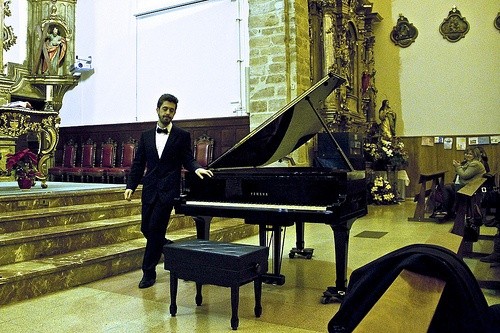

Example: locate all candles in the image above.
[46,84,54,100]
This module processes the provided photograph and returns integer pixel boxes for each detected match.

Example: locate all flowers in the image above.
[3,150,39,180]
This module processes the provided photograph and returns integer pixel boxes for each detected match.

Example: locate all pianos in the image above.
[173,73,368,304]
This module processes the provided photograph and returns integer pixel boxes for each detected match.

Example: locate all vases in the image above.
[18,178,32,190]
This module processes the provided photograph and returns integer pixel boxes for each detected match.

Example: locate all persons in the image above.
[379,100,396,142]
[43,27,67,76]
[124,94,213,288]
[441,145,490,222]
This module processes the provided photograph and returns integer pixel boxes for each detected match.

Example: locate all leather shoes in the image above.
[138,277,155,287]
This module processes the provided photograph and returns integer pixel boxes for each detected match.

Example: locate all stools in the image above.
[162,237,270,331]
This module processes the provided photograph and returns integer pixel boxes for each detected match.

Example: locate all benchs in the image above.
[352,169,500,333]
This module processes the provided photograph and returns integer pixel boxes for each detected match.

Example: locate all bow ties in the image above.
[157,128,168,135]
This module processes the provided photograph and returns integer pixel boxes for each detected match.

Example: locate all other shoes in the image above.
[439,212,454,222]
[435,203,451,211]
[480,252,500,263]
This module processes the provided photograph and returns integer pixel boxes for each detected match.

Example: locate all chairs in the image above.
[63,139,98,182]
[48,139,77,181]
[181,134,215,184]
[107,134,136,183]
[83,137,118,182]
